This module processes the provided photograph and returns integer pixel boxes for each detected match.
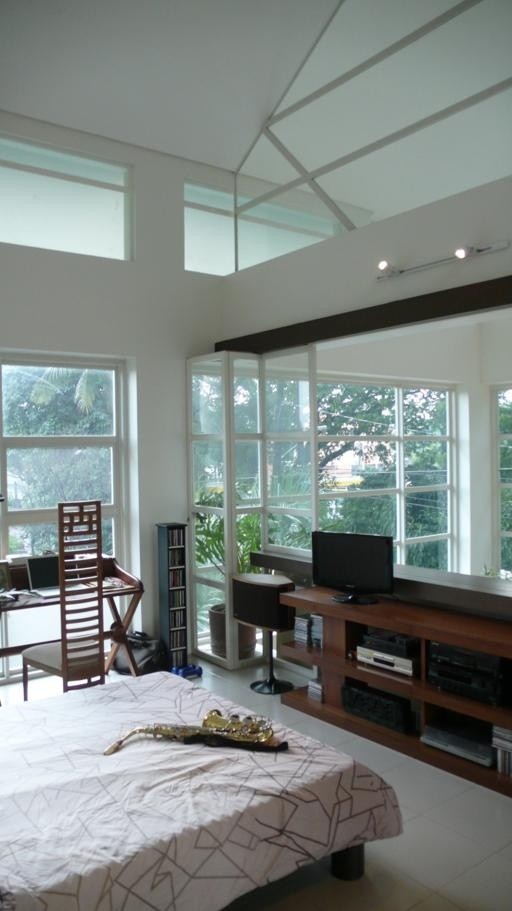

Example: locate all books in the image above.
[293,614,323,649]
[307,680,325,703]
[168,530,187,669]
[489,725,511,776]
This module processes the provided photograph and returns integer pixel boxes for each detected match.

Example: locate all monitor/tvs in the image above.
[312,531,394,605]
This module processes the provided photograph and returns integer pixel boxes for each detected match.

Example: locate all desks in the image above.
[0,551,144,707]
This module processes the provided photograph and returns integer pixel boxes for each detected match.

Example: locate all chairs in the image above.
[22,501,106,701]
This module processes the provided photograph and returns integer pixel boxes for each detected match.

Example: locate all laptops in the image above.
[25,553,90,599]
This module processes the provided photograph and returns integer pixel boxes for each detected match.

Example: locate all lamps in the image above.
[376,245,473,282]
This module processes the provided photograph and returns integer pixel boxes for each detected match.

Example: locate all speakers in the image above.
[231,575,295,633]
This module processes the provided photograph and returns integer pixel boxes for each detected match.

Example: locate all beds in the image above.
[1,671,403,910]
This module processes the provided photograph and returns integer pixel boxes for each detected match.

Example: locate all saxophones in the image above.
[103,709,289,756]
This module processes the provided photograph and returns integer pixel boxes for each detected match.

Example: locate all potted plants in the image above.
[192,487,280,660]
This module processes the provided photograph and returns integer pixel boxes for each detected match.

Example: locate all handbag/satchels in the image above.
[110,636,170,676]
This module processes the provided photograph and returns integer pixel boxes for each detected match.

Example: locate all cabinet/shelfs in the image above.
[279,586,512,797]
[155,522,188,673]
